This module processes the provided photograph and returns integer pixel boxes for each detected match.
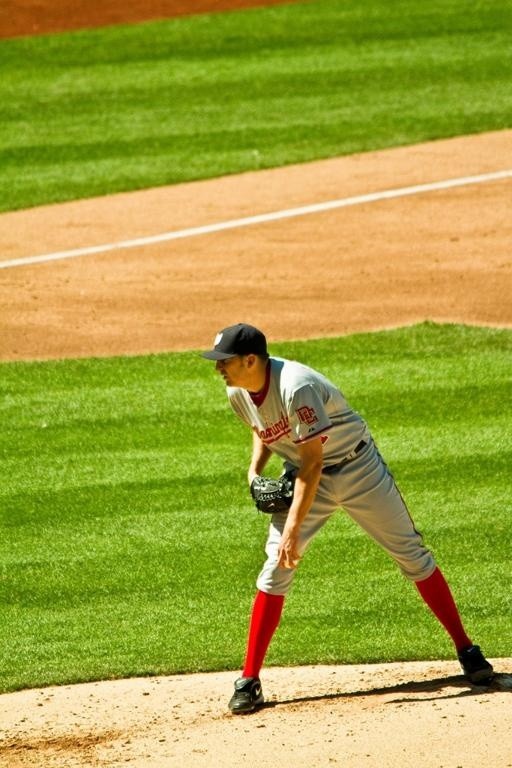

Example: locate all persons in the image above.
[199,322,492,714]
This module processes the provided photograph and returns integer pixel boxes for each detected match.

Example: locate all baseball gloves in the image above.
[250,478,292,513]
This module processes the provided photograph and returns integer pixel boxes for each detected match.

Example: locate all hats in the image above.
[198,324,267,360]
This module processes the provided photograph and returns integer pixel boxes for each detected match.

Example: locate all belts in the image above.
[318,439,365,469]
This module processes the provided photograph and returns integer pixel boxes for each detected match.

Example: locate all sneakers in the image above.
[457,643,496,689]
[228,679,266,715]
